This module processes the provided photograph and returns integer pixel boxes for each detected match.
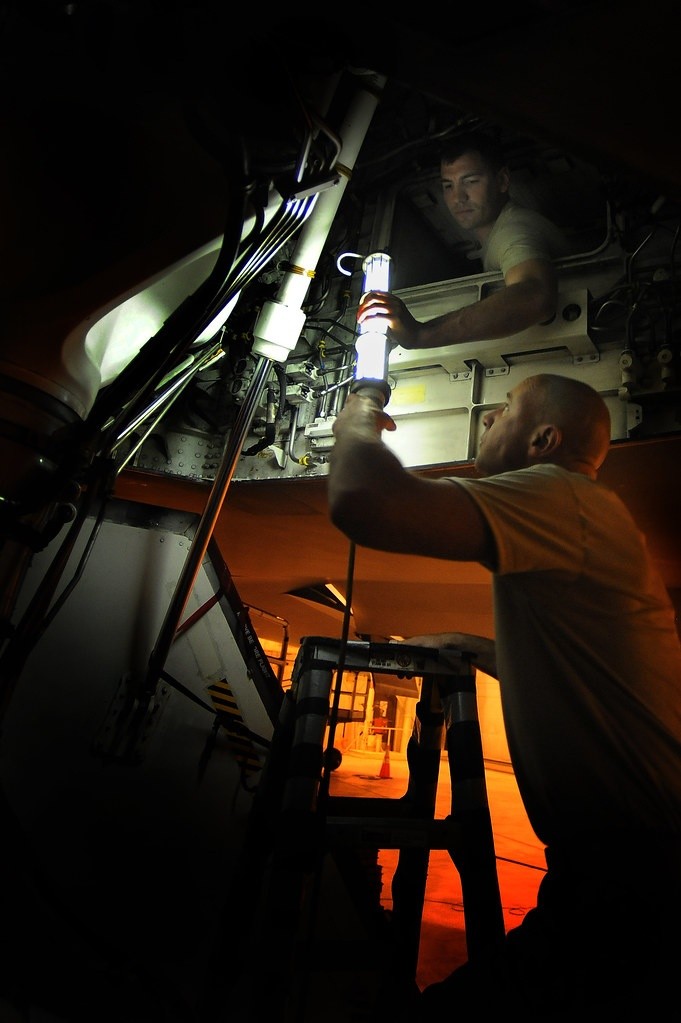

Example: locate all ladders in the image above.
[210,636,518,1023]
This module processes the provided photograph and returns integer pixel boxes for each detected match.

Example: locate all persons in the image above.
[327,372,680,1023]
[354,133,570,348]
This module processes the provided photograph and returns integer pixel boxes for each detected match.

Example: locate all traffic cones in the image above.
[376,753,393,780]
